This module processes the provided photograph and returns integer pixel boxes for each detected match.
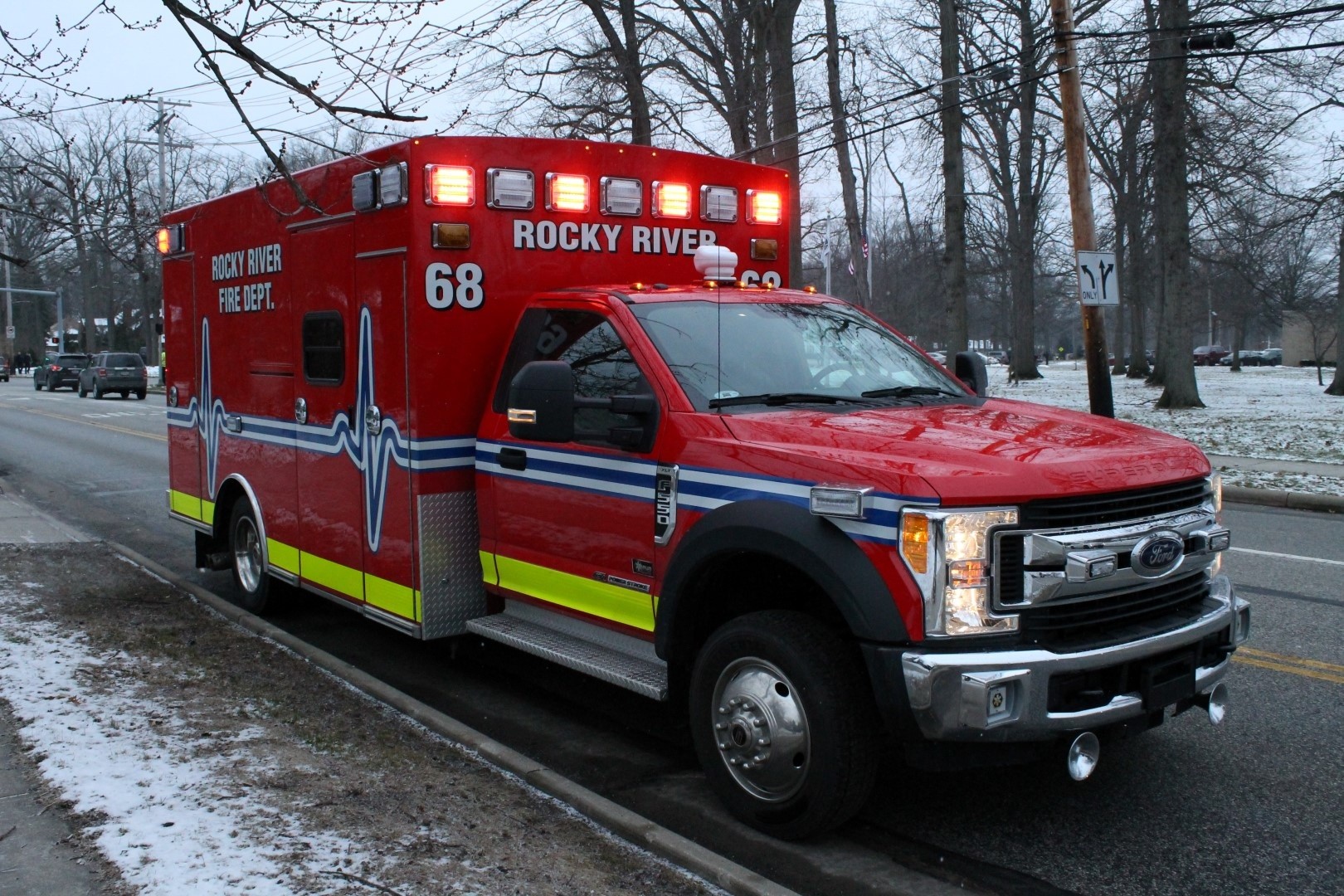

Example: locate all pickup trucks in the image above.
[1193,344,1232,366]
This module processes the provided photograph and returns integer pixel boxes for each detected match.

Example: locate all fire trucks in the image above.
[158,133,1258,849]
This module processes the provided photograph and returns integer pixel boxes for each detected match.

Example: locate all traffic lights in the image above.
[52,337,59,344]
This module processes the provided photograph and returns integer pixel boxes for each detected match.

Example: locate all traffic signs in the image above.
[1076,250,1122,309]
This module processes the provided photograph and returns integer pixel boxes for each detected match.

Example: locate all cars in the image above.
[1259,348,1283,367]
[0,356,10,383]
[924,349,1008,366]
[1219,349,1261,367]
[1107,348,1156,367]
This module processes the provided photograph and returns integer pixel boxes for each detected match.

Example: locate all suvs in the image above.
[76,351,148,400]
[33,352,93,392]
